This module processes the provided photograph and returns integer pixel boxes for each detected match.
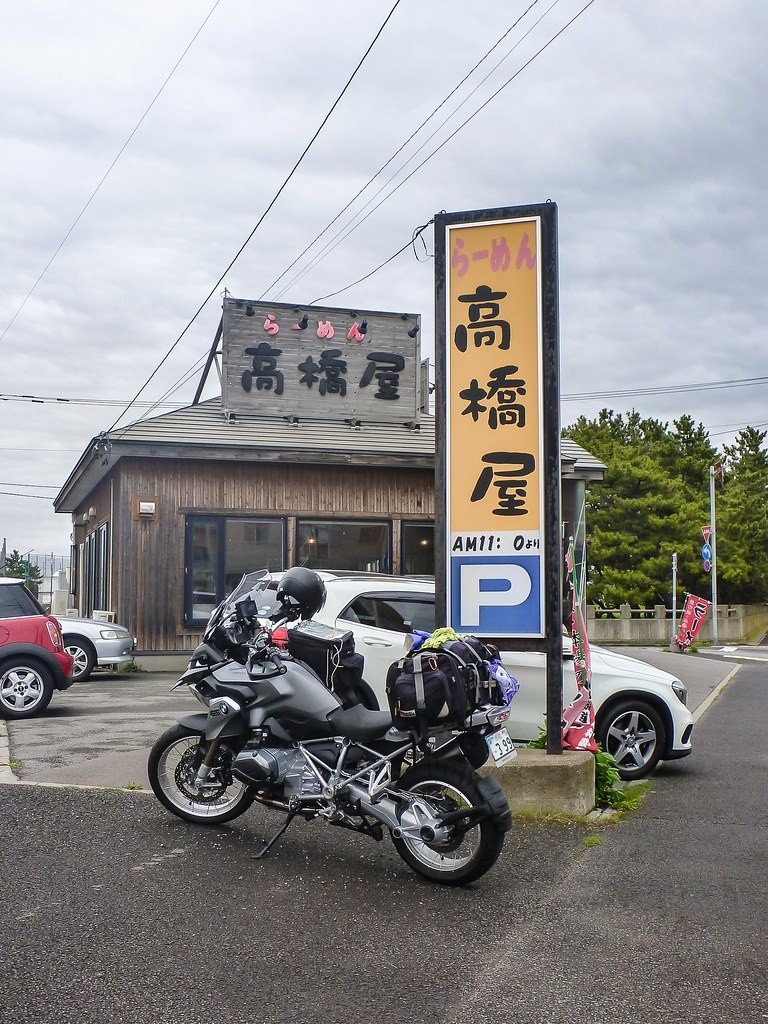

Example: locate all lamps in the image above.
[293,308,310,329]
[401,313,420,338]
[350,310,369,334]
[428,382,435,394]
[236,300,256,316]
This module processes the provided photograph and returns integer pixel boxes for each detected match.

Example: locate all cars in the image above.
[51,615,138,684]
[192,589,229,621]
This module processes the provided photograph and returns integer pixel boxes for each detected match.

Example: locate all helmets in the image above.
[276,566,326,621]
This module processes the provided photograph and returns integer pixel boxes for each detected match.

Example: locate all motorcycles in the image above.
[147,568,519,889]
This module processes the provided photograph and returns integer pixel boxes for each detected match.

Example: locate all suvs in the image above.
[0,576,74,720]
[199,568,696,783]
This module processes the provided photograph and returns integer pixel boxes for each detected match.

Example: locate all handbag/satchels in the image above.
[385,634,501,742]
